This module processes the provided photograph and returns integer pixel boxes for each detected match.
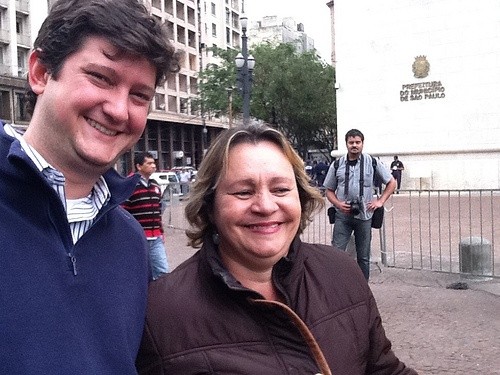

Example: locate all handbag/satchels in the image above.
[328,207,337,224]
[371,205,385,229]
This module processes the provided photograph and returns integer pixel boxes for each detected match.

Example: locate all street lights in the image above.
[234,14,256,125]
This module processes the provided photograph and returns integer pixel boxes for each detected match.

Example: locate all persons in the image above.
[178,168,190,201]
[0,0,184,375]
[324,129,397,284]
[136,122,418,375]
[302,156,404,194]
[119,151,172,280]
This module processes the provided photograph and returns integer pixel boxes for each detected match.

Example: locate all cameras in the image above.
[344,199,360,215]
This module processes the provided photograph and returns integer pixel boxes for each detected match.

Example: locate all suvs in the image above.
[148,165,199,194]
